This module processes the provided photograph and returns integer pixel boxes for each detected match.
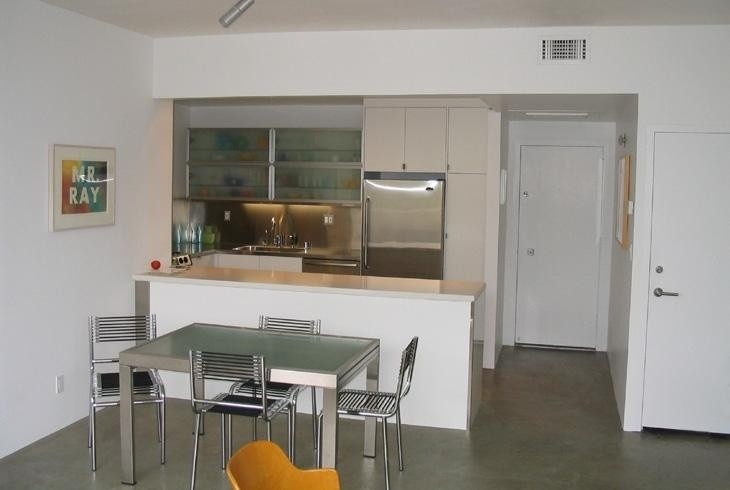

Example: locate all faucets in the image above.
[273,234,285,248]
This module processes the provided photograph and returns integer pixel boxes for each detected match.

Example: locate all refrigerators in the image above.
[362,177,445,279]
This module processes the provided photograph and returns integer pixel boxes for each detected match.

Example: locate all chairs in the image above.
[188,317,418,490]
[89,315,166,470]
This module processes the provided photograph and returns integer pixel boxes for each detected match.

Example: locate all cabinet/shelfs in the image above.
[363,99,486,171]
[443,174,485,343]
[173,103,363,207]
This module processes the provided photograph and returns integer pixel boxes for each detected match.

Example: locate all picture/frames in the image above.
[48,143,116,230]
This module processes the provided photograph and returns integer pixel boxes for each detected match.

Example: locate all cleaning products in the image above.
[201,225,216,248]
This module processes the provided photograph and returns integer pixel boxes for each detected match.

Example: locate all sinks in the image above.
[232,244,309,254]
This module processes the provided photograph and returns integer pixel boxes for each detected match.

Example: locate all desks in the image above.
[117,323,380,490]
